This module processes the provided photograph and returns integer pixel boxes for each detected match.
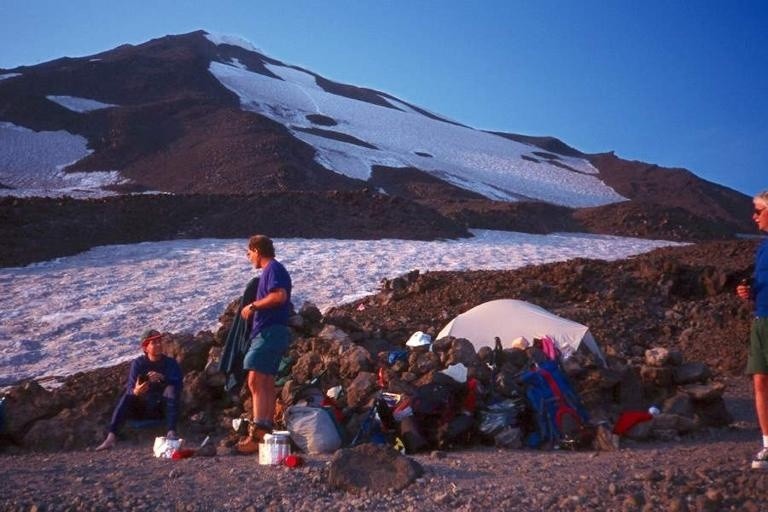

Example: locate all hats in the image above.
[139,329,161,344]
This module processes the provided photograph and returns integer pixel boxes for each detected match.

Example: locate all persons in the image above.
[232,233,293,458]
[733,189,767,478]
[93,327,184,453]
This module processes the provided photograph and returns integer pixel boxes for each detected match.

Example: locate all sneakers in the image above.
[752,446,768,471]
[95,437,117,451]
[231,422,271,454]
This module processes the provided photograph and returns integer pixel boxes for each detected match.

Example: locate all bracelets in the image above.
[247,302,258,312]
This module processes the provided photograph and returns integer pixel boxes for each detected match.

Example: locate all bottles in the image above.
[270,433,288,465]
[740,277,753,303]
